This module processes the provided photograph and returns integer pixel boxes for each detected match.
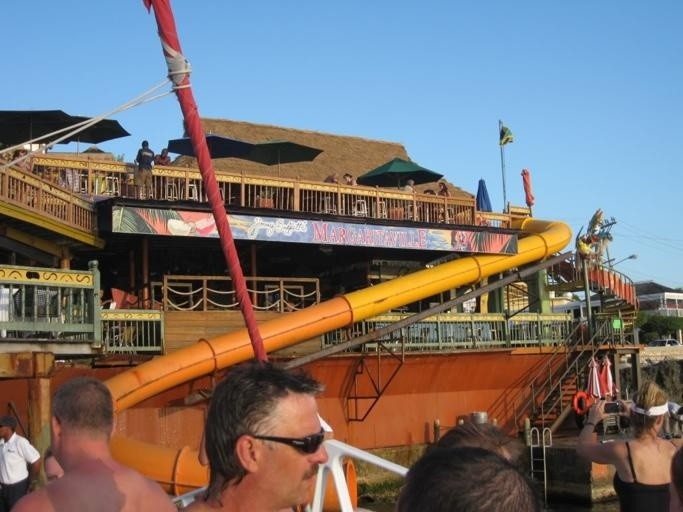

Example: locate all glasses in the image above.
[255,426,326,455]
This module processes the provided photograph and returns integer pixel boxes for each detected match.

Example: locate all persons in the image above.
[135,140,154,200]
[576,380,683,512]
[322,173,450,223]
[43,446,64,483]
[0,415,41,509]
[437,423,531,477]
[396,447,541,512]
[13,272,115,327]
[181,359,329,512]
[154,149,171,199]
[9,375,178,512]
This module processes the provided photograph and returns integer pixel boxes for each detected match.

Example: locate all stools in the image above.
[319,194,455,226]
[77,172,201,204]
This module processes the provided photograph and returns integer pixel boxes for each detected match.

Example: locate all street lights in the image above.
[608,252,641,267]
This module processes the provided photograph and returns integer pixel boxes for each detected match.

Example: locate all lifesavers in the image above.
[573,391,590,416]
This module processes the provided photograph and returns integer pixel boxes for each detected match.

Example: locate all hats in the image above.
[628,399,670,417]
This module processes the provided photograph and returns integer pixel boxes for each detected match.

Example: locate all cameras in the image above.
[605,403,623,413]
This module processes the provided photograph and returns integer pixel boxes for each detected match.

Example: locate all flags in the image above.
[500,126,514,146]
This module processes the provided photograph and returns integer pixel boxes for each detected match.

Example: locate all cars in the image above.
[594,337,630,344]
[645,338,680,347]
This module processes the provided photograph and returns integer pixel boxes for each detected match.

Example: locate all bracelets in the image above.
[585,423,597,431]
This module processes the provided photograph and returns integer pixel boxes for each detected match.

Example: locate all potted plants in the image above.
[255,190,274,209]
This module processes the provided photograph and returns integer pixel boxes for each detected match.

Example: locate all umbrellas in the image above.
[601,355,615,400]
[0,109,70,152]
[521,169,534,217]
[356,157,444,190]
[588,357,602,404]
[66,116,132,158]
[235,139,325,180]
[476,176,493,212]
[168,129,257,163]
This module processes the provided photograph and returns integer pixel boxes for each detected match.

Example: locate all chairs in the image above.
[370,318,530,353]
